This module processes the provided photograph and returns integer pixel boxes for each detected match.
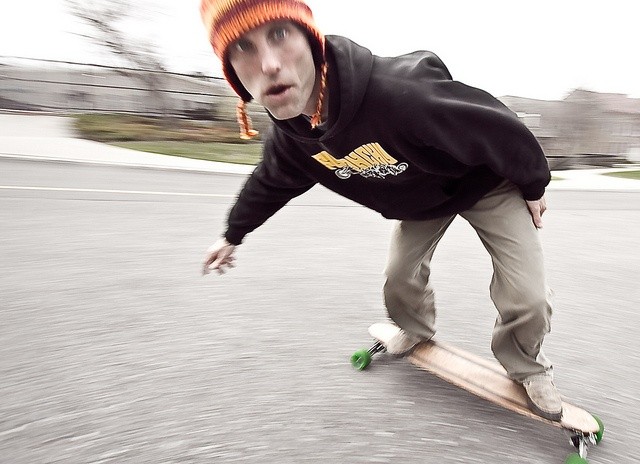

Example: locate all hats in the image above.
[198,0,328,139]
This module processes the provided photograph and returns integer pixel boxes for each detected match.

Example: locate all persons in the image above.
[200,0,565,420]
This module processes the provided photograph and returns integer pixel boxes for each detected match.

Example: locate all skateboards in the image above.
[351,321,604,464]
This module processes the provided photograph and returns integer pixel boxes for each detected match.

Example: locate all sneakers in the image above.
[385,330,420,357]
[523,374,563,421]
